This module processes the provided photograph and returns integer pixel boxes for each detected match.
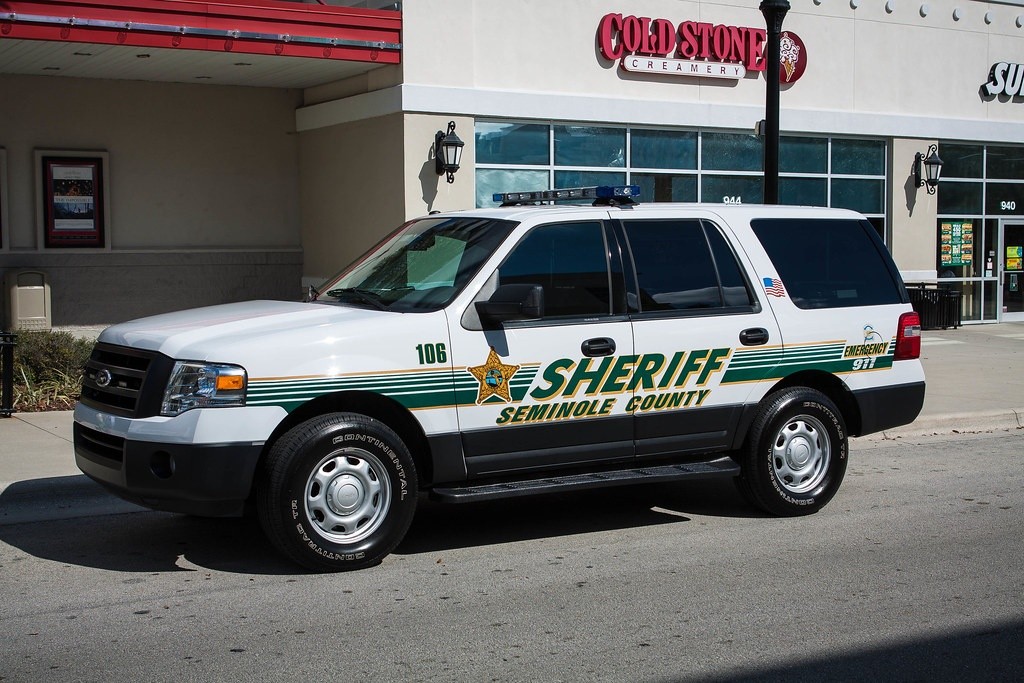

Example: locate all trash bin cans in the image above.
[3,267,52,334]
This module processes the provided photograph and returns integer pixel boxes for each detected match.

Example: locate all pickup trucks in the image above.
[71,186,926,572]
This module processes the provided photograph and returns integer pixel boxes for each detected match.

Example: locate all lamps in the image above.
[915,144,944,195]
[435,120,465,184]
